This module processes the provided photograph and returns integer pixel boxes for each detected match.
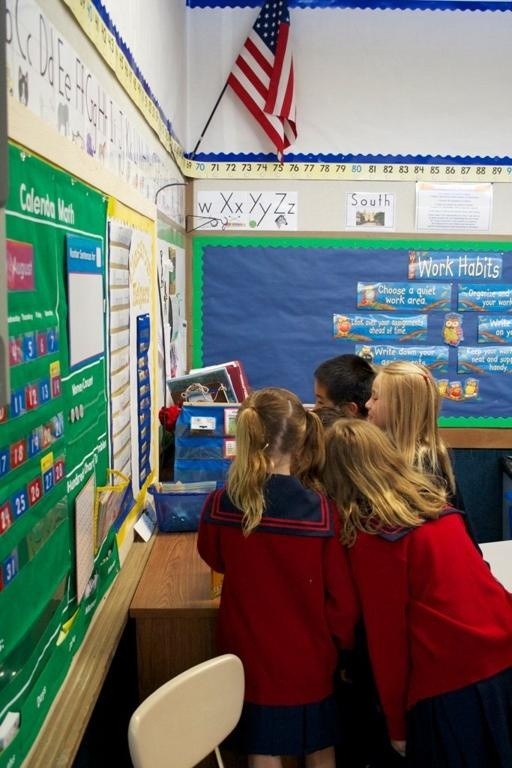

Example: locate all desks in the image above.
[131,534,220,702]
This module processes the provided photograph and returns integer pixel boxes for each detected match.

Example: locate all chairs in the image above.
[126,655,247,768]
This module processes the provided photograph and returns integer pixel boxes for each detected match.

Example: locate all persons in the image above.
[363,360,484,566]
[297,405,357,490]
[315,418,512,768]
[308,355,372,427]
[195,387,361,767]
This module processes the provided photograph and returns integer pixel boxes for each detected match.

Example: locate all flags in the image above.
[225,1,298,166]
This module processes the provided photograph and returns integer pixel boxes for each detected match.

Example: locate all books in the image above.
[166,359,249,406]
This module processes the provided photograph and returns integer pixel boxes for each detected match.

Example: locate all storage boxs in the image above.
[147,481,228,532]
